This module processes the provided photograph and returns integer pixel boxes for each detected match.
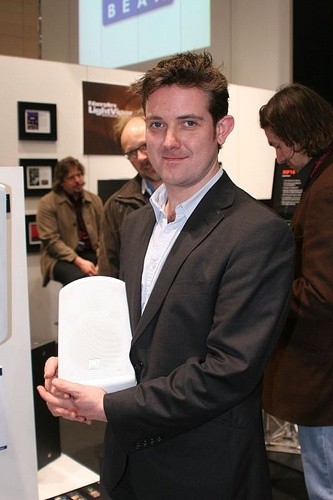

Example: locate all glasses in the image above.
[124,144,146,160]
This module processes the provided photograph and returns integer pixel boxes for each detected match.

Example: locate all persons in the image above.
[38,157,104,288]
[37,50,295,500]
[97,113,164,326]
[259,86,333,500]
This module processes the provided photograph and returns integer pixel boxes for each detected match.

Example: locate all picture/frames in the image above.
[25,214,41,252]
[18,158,58,197]
[17,101,58,141]
